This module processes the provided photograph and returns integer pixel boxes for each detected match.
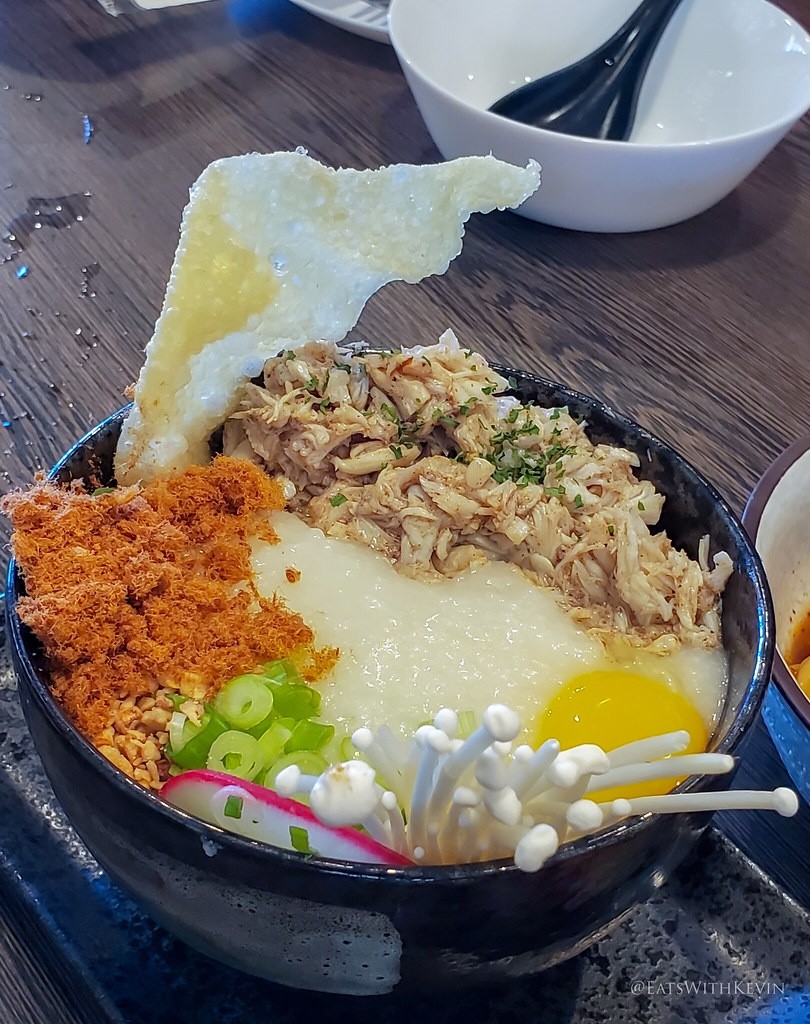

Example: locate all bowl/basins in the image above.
[741,434,810,806]
[385,0,810,233]
[4,350,775,1000]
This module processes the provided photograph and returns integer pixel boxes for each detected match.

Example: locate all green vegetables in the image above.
[283,346,646,539]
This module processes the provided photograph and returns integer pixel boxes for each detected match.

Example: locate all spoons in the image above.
[489,0,680,142]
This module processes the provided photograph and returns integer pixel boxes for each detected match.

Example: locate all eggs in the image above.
[518,665,716,814]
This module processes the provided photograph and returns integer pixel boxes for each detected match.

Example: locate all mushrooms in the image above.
[278,703,798,873]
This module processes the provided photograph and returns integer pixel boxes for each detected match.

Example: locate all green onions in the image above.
[165,661,384,853]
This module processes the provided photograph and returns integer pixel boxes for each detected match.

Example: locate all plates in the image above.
[291,0,391,48]
[0,592,810,1024]
